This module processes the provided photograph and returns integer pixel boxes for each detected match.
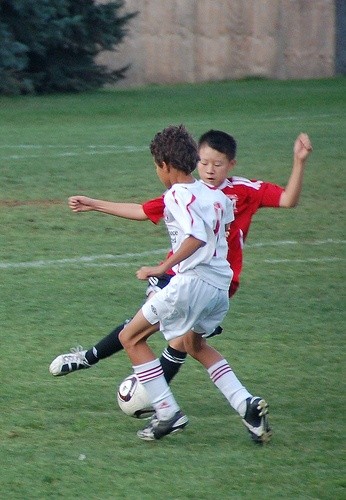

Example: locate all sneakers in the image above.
[137,409,189,441]
[241,396,272,445]
[48,345,92,377]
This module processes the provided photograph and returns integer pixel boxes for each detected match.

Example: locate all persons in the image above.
[48,129,314,386]
[118,123,272,444]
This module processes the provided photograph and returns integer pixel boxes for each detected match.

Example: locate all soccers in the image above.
[116,373,156,419]
